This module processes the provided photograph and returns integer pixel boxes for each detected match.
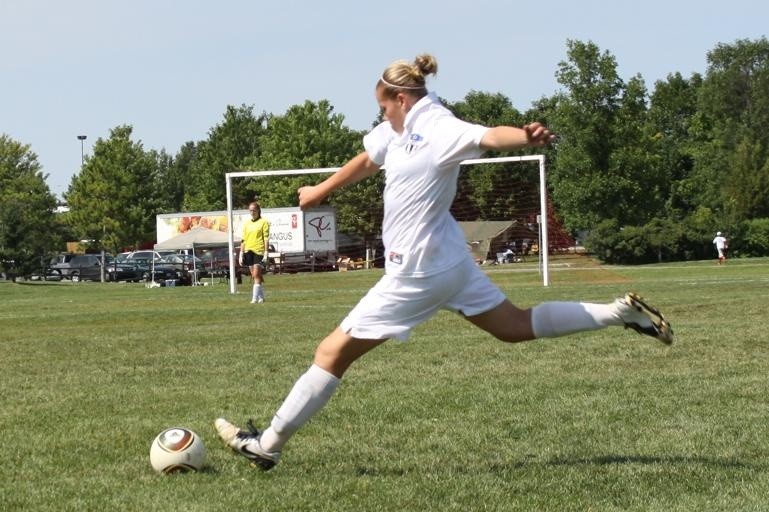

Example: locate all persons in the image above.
[237,202,271,306]
[211,55,676,471]
[712,231,729,266]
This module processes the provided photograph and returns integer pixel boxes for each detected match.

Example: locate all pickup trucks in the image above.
[267,244,305,273]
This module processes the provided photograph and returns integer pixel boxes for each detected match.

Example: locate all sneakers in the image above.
[608,293,674,346]
[214,417,281,473]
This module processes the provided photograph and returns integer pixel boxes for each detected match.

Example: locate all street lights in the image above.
[77,136,87,165]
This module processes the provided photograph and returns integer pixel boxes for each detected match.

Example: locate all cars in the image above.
[45,249,275,283]
[303,257,339,272]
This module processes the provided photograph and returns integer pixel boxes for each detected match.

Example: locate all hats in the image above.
[717,231,721,236]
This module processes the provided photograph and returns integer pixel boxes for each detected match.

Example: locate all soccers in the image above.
[149,427,205,474]
